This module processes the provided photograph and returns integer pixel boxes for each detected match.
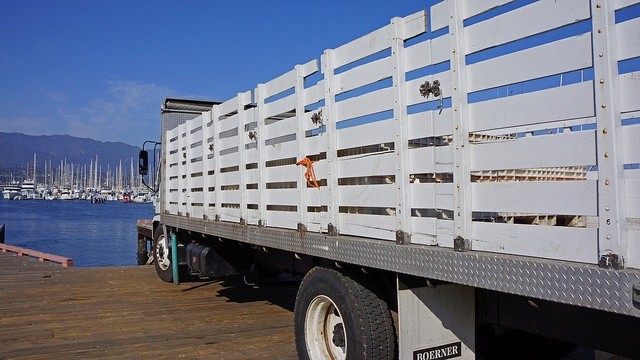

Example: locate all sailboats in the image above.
[2,151,154,204]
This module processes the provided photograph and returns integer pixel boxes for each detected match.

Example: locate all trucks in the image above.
[139,0,639,360]
